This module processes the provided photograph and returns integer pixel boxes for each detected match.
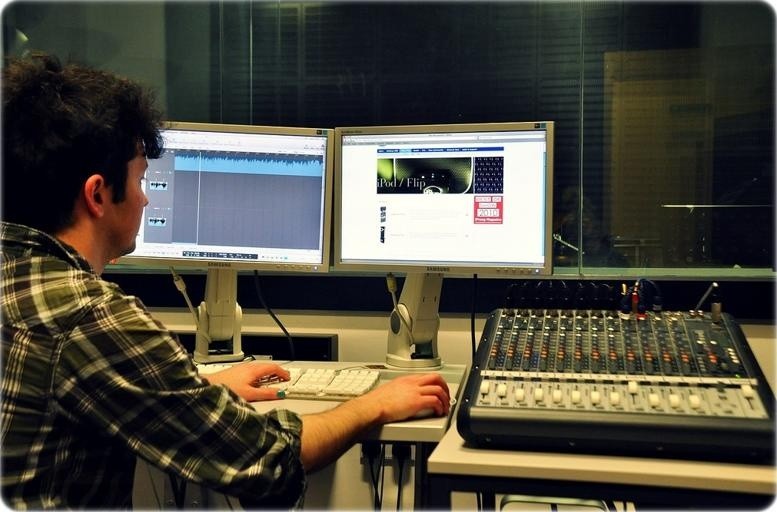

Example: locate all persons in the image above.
[0,57,447,508]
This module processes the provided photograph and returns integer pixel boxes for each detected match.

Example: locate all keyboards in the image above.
[192,366,380,399]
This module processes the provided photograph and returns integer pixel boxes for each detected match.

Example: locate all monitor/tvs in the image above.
[86,120,332,364]
[334,121,555,370]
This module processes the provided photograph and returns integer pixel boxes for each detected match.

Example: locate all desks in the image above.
[164,359,467,512]
[425,319,776,512]
